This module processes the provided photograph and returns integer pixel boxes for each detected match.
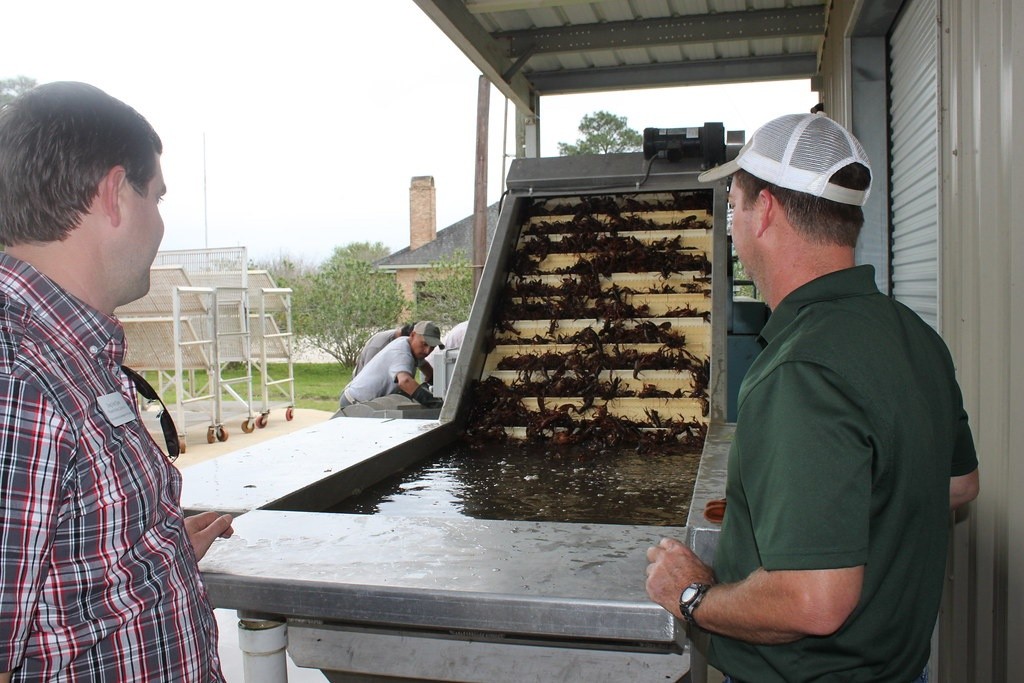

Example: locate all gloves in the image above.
[429,376,433,385]
[411,385,444,408]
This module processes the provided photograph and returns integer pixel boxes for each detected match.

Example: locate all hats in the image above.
[412,320,445,350]
[698,110,872,206]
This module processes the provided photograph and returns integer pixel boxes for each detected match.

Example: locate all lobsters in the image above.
[454,191,712,460]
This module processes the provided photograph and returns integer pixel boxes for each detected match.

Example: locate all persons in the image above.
[646,111,980,683]
[0,82,234,683]
[340,319,468,406]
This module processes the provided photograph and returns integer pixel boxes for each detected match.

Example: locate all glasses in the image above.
[120,365,180,464]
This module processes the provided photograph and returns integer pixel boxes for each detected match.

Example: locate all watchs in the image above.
[679,582,717,635]
